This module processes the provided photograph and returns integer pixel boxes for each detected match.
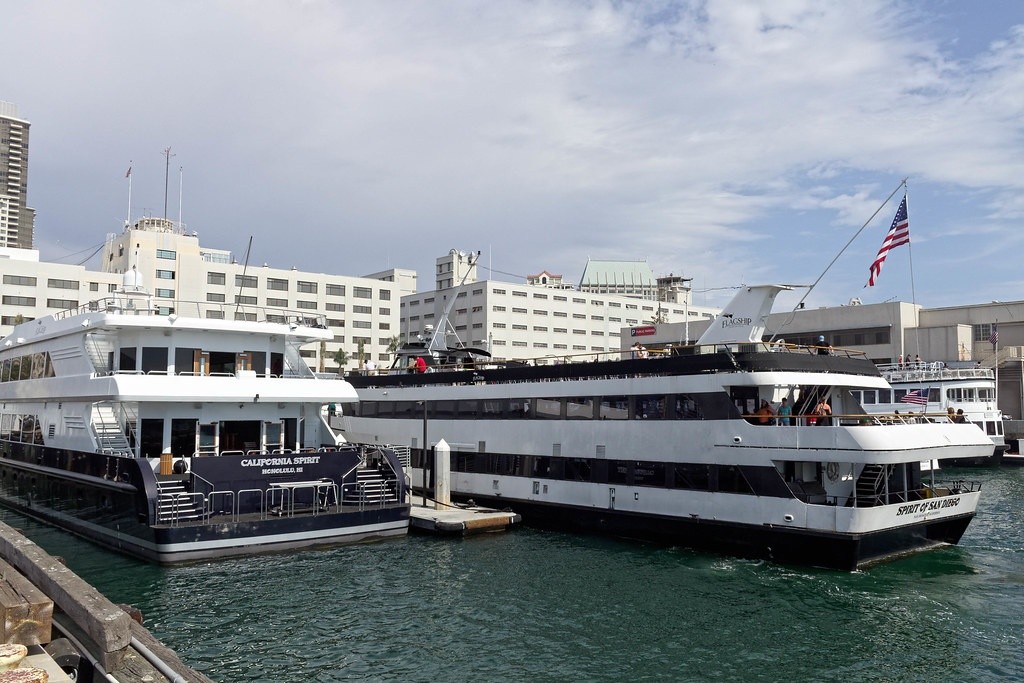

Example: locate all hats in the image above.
[819,335,825,342]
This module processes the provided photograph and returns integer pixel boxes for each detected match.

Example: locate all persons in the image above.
[943,363,951,370]
[813,335,834,355]
[755,400,774,426]
[777,398,792,426]
[413,356,426,387]
[943,407,965,424]
[974,361,981,369]
[364,359,377,389]
[816,397,832,426]
[879,409,917,424]
[898,353,921,369]
[630,342,649,378]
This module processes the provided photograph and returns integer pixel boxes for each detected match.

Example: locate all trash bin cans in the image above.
[160,452,173,476]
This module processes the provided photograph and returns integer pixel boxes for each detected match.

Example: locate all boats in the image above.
[1,247,1011,566]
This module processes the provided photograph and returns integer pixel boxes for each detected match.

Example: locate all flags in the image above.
[901,388,929,405]
[988,325,999,345]
[862,193,909,288]
